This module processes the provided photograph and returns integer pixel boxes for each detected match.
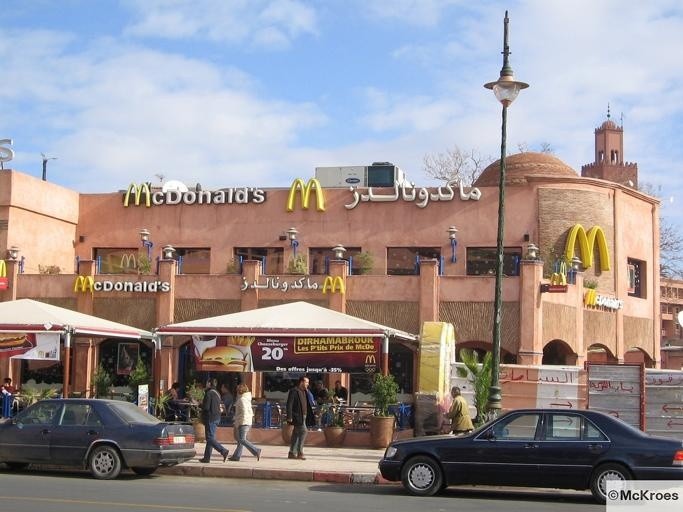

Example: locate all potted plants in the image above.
[184,378,206,443]
[280,408,294,445]
[366,371,399,450]
[19,384,61,424]
[313,388,346,448]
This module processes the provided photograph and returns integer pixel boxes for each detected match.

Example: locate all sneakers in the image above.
[288,452,297,458]
[199,458,210,463]
[224,450,229,462]
[256,449,261,461]
[228,458,239,461]
[297,454,306,460]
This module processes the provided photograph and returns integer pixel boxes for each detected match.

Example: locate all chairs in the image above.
[51,409,75,426]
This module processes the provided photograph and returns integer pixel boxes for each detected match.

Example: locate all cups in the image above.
[191,335,217,358]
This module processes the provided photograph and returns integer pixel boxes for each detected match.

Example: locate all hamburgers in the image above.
[0,334,26,347]
[201,346,247,371]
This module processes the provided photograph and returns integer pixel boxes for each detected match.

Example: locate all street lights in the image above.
[479,11,532,434]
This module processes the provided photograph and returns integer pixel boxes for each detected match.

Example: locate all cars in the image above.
[374,406,683,506]
[1,396,198,481]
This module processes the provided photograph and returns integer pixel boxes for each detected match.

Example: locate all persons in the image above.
[442,386,473,434]
[198,376,228,462]
[220,383,233,416]
[225,383,261,462]
[162,382,187,422]
[332,380,347,404]
[285,376,316,461]
[313,380,333,415]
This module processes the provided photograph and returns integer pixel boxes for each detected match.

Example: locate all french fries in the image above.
[227,335,255,346]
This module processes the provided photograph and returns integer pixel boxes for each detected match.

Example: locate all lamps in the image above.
[331,244,346,260]
[9,245,19,260]
[569,257,582,270]
[524,244,538,260]
[445,225,456,246]
[162,244,176,258]
[285,227,297,246]
[138,229,152,247]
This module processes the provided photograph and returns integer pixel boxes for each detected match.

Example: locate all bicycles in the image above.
[425,411,495,437]
